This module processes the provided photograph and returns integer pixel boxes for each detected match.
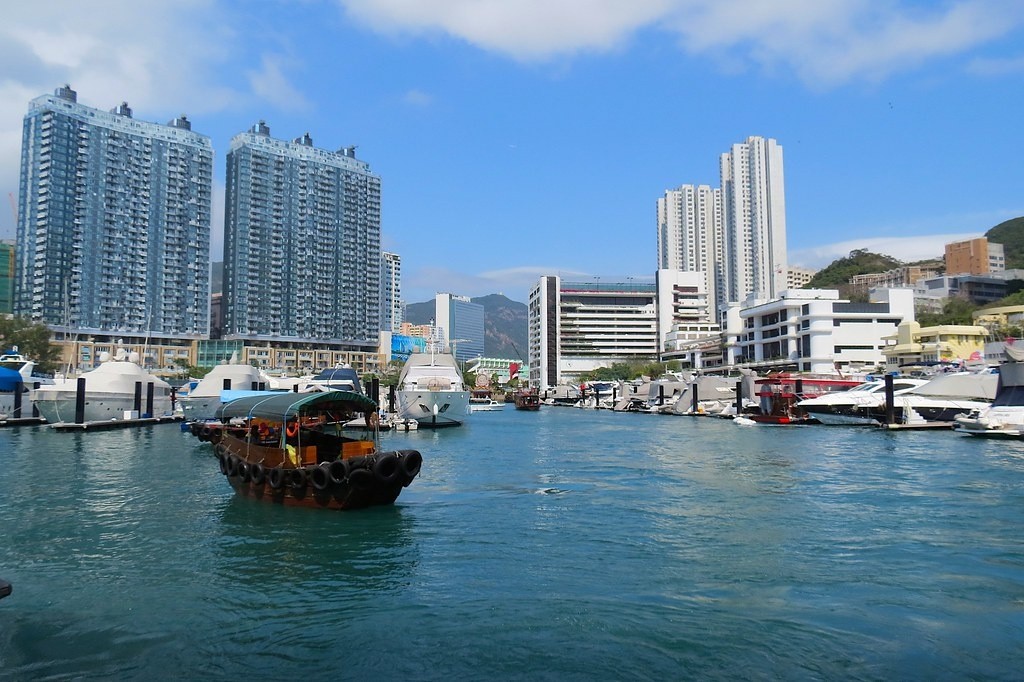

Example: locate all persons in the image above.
[245,419,298,446]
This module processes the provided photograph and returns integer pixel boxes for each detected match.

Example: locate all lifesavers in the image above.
[251,464,265,485]
[286,423,299,438]
[329,460,348,483]
[219,455,229,475]
[402,451,423,477]
[227,456,239,477]
[269,468,285,489]
[237,462,251,483]
[213,428,222,437]
[202,427,211,436]
[347,468,375,492]
[375,454,401,479]
[289,470,307,489]
[310,467,329,490]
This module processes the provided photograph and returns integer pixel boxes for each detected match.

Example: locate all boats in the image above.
[951,344,1024,441]
[0,341,57,425]
[210,390,422,513]
[854,371,999,434]
[515,393,541,412]
[548,370,852,422]
[392,318,472,428]
[173,364,392,432]
[504,387,520,403]
[789,378,931,426]
[27,360,175,430]
[469,395,506,411]
